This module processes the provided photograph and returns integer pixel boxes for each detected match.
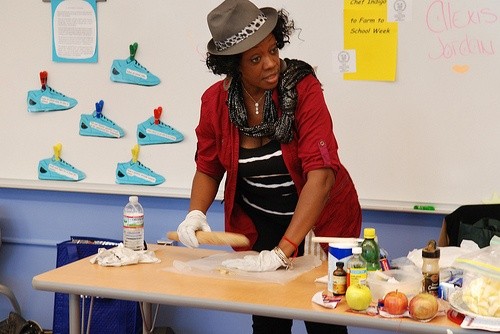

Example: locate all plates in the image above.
[460,316,500,332]
[449,289,500,324]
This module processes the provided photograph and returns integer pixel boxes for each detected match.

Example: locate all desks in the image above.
[31,242,500,334]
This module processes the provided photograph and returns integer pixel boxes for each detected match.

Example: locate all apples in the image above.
[346,284,371,310]
[384,288,408,314]
[409,293,437,319]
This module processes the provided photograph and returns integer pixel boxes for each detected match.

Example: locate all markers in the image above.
[413,205,435,210]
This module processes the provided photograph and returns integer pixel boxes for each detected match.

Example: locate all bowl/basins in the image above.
[368,269,413,303]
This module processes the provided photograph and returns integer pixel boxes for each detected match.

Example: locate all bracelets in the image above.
[283,236,298,249]
[274,246,294,271]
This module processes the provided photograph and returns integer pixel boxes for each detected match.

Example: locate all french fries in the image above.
[462,276,500,319]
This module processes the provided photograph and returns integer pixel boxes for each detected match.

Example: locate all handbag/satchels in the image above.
[52,235,147,334]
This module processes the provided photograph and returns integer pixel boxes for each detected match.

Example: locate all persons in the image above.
[177,0,361,334]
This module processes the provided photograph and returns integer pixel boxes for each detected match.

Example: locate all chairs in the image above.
[437,203,500,249]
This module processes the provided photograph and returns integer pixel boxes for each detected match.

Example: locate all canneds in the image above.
[379,258,390,271]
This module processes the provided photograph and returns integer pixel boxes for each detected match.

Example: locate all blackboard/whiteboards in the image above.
[1,0,500,215]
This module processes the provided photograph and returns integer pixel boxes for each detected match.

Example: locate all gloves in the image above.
[177,210,212,248]
[224,250,286,271]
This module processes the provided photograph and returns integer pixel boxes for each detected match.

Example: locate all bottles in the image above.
[360,227,381,275]
[123,195,144,252]
[421,240,440,299]
[414,205,436,210]
[332,246,367,296]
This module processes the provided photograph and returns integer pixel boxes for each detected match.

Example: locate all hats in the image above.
[206,0,278,56]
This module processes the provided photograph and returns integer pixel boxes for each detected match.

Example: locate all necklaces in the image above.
[241,82,268,115]
